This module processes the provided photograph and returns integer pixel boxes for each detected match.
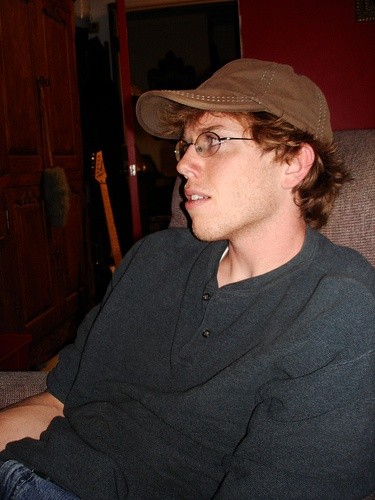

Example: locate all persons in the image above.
[1,57,375,500]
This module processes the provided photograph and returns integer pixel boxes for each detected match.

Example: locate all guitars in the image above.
[91,151,124,272]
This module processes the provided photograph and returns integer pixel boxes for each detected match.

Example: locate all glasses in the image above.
[174,132,256,163]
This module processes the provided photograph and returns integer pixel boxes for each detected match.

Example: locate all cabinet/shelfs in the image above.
[1,331,33,372]
[0,0,93,367]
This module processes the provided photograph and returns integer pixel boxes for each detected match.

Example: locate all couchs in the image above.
[0,125,374,419]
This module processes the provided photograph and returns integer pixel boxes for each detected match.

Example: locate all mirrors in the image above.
[114,0,243,244]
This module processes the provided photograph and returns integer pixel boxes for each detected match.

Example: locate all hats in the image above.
[135,59,333,150]
